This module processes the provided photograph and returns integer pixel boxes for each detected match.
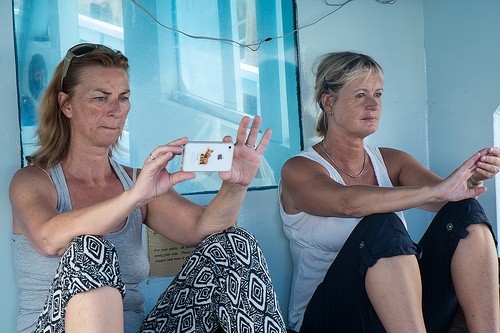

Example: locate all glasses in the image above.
[60,43,116,91]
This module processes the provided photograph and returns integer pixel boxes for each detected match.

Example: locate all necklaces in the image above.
[320,139,366,178]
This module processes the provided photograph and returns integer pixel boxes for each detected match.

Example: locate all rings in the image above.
[150,154,155,160]
[245,143,256,149]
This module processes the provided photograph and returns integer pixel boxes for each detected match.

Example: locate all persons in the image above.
[277,51,500,333]
[9,44,287,333]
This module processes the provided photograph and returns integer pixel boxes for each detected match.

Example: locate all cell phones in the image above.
[180,141,235,172]
